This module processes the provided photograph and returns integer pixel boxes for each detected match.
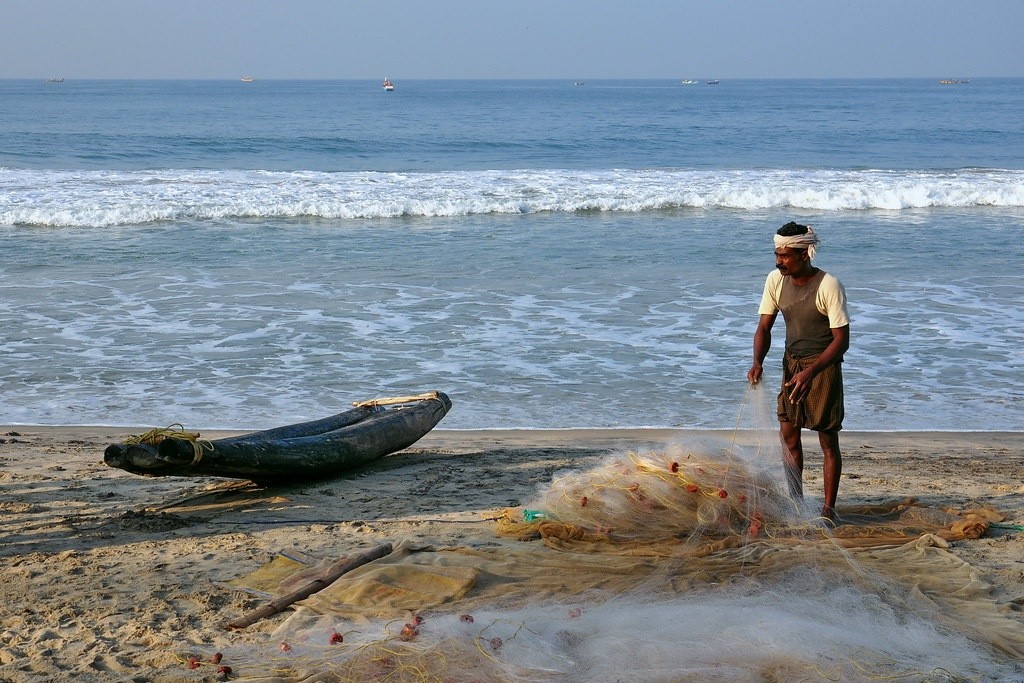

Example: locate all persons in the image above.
[747,221,849,527]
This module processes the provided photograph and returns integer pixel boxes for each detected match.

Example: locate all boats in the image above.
[103,391,452,490]
